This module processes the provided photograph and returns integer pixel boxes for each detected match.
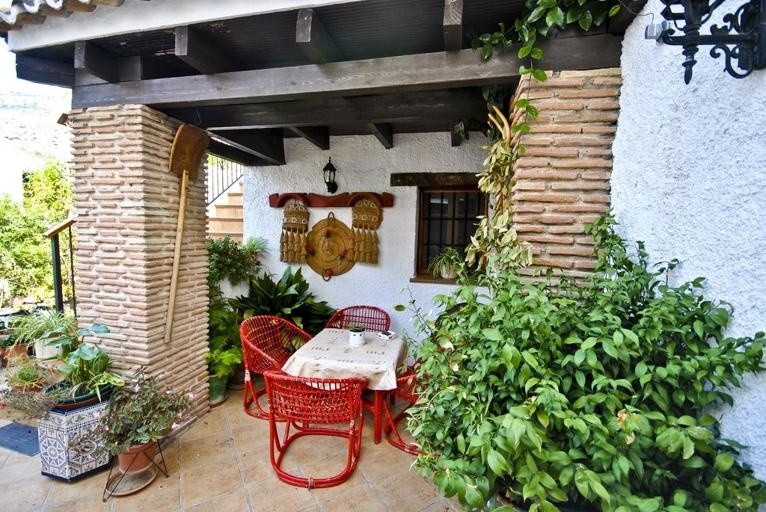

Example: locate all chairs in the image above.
[263,370,370,489]
[325,305,391,331]
[382,339,468,456]
[240,315,324,421]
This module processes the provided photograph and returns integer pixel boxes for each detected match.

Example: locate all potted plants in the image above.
[82,364,196,476]
[426,246,465,280]
[39,324,125,410]
[14,317,29,367]
[12,308,79,361]
[208,308,242,406]
[7,363,47,393]
[230,309,256,385]
[348,321,367,347]
[0,334,15,368]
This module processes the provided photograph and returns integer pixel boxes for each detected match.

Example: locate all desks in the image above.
[280,327,407,445]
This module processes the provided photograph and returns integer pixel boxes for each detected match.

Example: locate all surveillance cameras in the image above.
[57,113,69,126]
[645,19,676,46]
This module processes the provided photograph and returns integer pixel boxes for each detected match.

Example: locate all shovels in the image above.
[164,123,209,343]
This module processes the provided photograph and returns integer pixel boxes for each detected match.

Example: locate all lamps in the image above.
[322,156,338,194]
